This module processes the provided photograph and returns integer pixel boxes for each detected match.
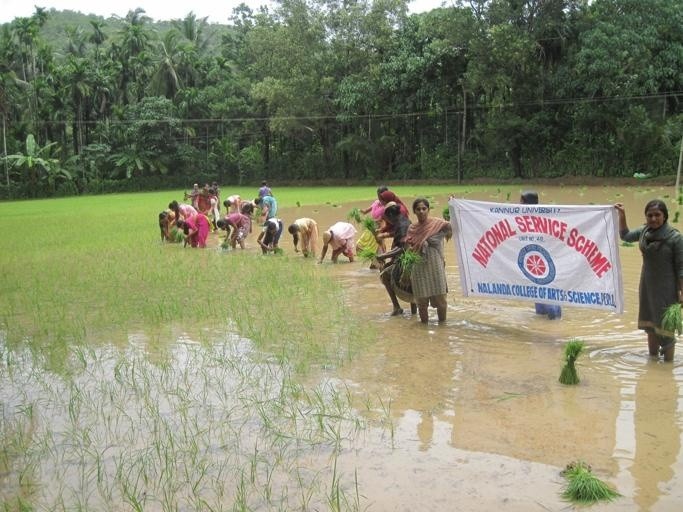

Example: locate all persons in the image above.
[257,180,273,210]
[216,213,251,249]
[256,218,283,256]
[254,196,276,221]
[176,213,211,249]
[223,194,240,216]
[157,210,184,244]
[287,218,318,257]
[168,201,198,234]
[182,180,220,233]
[317,222,357,264]
[613,199,682,360]
[403,194,454,324]
[358,186,419,316]
[518,191,560,320]
[238,199,253,216]
[624,358,681,512]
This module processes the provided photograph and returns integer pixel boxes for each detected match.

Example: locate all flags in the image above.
[448,336,623,475]
[448,197,627,315]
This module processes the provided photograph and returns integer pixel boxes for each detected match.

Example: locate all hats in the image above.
[323,231,331,246]
[380,191,395,203]
[384,201,396,214]
